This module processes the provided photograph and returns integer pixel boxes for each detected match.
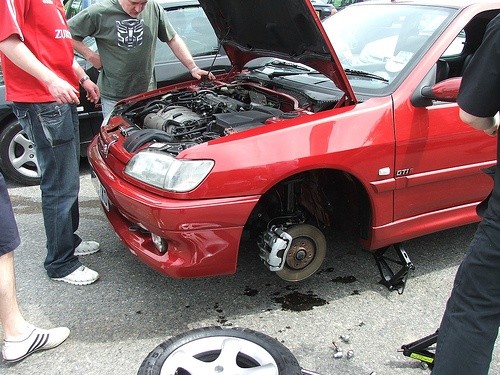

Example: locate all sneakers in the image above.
[52,264,99,285]
[73,241,101,255]
[2,326,70,363]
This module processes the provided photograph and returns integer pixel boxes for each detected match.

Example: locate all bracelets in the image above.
[190,66,199,73]
[79,75,90,84]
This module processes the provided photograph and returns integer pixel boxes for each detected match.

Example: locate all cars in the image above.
[0,0,338,187]
[355,13,467,67]
[86,0,500,289]
[63,0,103,20]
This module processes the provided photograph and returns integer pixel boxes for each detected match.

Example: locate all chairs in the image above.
[158,15,188,52]
[414,36,450,83]
[187,18,215,52]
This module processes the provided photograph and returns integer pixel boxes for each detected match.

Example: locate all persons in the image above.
[430,15,500,375]
[66,0,216,120]
[0,173,70,363]
[0,0,101,285]
